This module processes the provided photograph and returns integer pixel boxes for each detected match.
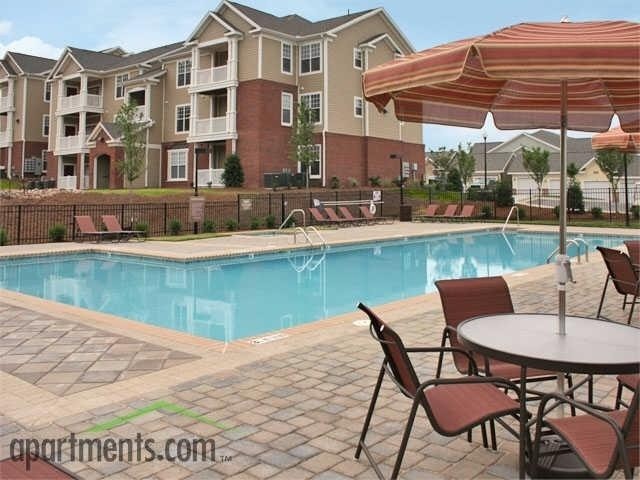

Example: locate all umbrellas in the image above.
[590,124,640,153]
[361,15,640,418]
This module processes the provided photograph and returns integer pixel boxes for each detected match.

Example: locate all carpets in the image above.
[0,452,85,480]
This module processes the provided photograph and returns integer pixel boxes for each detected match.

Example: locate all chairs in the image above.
[308,206,394,230]
[354,276,639,480]
[594,240,640,325]
[74,214,147,244]
[412,204,487,223]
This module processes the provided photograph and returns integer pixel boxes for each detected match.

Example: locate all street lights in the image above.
[190,147,205,234]
[482,133,488,189]
[391,155,404,205]
